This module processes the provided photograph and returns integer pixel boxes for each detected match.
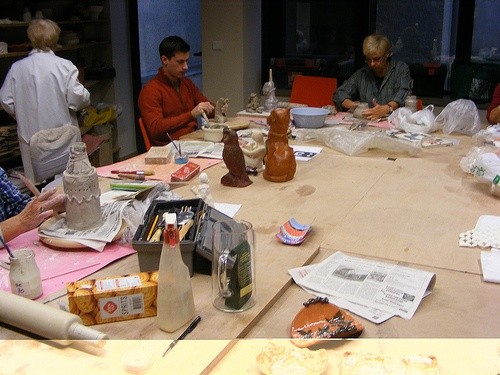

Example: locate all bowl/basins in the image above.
[289,107,331,128]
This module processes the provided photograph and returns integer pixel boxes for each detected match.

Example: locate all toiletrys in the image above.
[156,212,196,333]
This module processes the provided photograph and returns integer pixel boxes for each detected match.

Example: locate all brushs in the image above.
[111,170,154,176]
[16,172,60,220]
[201,108,213,128]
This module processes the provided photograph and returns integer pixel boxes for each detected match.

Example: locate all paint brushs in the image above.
[166,132,179,152]
[147,209,160,240]
[179,143,182,156]
[0,235,14,258]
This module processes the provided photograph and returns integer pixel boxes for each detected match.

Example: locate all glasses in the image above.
[365,52,386,65]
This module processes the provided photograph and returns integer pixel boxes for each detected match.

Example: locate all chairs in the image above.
[290,75,337,107]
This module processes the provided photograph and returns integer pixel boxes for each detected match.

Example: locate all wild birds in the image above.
[220,128,253,189]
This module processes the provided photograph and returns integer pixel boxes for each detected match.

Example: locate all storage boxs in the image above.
[67,271,158,326]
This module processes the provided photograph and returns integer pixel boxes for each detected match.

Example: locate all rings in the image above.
[40,207,44,212]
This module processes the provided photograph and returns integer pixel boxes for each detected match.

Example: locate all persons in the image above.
[0,19,91,196]
[333,34,411,121]
[486,82,500,124]
[0,166,60,252]
[140,35,228,146]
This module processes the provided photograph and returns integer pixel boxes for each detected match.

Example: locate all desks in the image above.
[0,113,500,375]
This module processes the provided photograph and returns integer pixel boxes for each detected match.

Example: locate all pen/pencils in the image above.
[162,316,201,354]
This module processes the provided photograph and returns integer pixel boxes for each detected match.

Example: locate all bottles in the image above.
[0,290,109,346]
[9,248,43,299]
[405,95,417,113]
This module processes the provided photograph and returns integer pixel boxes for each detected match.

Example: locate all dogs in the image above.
[263,107,296,183]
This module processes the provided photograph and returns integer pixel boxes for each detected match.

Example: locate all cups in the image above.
[200,122,224,142]
[210,219,257,314]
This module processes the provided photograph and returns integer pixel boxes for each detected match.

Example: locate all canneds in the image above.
[8,249,43,299]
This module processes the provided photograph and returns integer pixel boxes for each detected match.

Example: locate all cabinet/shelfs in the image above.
[0,0,111,88]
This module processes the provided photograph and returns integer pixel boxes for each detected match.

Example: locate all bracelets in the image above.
[386,103,393,114]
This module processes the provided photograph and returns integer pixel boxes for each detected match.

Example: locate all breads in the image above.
[290,298,364,347]
